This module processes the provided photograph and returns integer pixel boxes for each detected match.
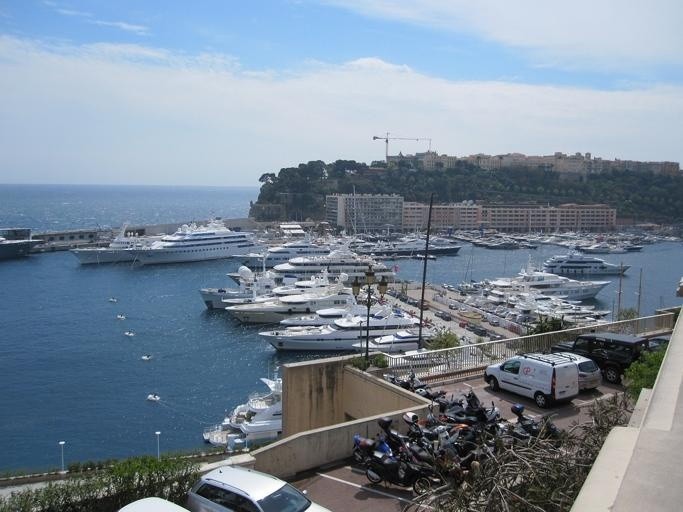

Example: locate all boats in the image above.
[0,237,44,259]
[457,226,682,327]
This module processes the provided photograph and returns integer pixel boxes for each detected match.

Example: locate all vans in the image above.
[121,463,339,512]
[483,352,581,407]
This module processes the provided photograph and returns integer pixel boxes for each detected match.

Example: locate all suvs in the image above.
[551,331,649,386]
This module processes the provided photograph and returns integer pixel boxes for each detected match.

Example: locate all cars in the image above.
[552,352,602,392]
[646,335,672,354]
[386,285,539,351]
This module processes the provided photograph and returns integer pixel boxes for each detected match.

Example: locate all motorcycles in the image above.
[353,365,563,497]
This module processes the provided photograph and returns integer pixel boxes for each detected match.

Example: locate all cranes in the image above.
[372,132,420,162]
